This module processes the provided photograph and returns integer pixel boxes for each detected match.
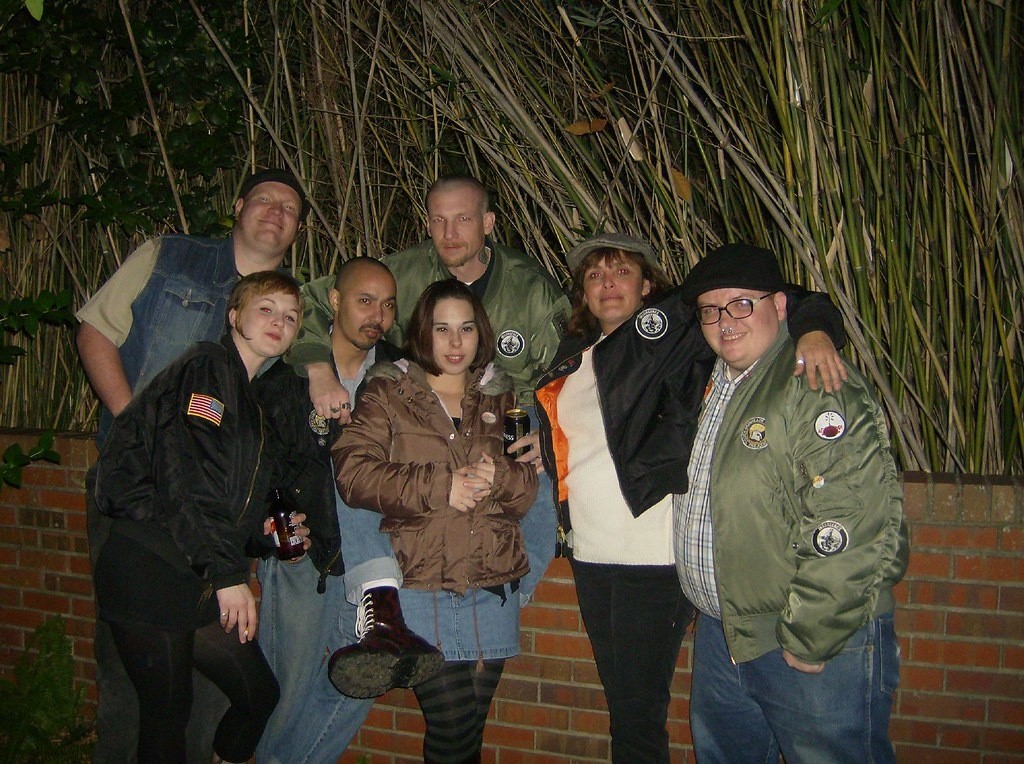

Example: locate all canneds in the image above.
[505,409,530,459]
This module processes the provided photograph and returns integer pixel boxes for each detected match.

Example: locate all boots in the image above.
[329,586,445,699]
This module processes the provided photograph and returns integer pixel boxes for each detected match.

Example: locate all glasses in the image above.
[695,293,777,325]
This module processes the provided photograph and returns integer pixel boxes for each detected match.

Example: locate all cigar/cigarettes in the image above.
[243,627,249,639]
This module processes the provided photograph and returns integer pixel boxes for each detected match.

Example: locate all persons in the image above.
[75,170,537,764]
[539,235,847,763]
[672,242,910,763]
[286,174,572,698]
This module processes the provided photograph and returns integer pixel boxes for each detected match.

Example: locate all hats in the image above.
[682,243,785,305]
[239,169,310,222]
[565,232,655,274]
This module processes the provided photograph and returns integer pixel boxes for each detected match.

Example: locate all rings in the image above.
[219,612,228,616]
[490,461,494,464]
[796,357,805,364]
[488,482,492,490]
[330,407,340,413]
[342,403,351,409]
